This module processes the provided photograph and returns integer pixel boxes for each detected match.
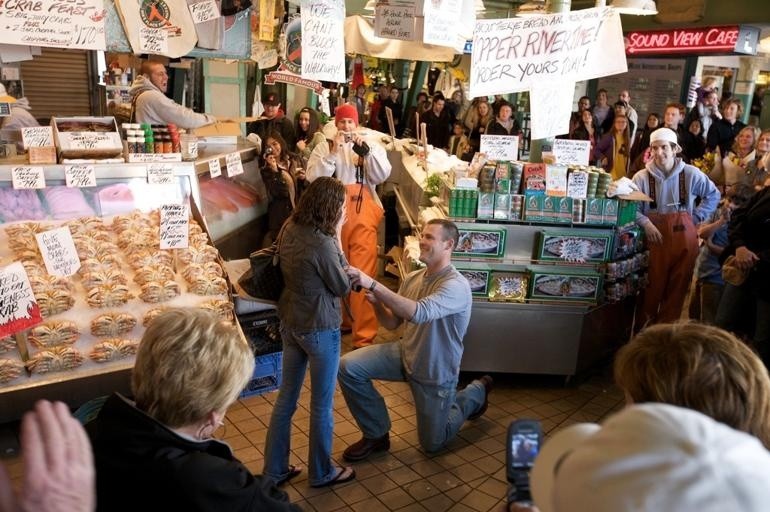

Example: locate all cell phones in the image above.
[505,420,543,512]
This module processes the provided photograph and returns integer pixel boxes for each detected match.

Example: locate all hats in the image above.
[335,105,359,128]
[650,127,683,154]
[261,92,280,106]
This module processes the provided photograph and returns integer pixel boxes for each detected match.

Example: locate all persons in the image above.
[0,399,98,512]
[127,61,217,130]
[336,218,491,463]
[75,307,306,512]
[0,83,41,129]
[264,174,364,487]
[247,77,770,372]
[522,321,770,511]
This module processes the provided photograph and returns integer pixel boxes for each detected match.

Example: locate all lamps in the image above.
[474,0,486,14]
[515,0,553,18]
[363,0,377,10]
[608,0,658,17]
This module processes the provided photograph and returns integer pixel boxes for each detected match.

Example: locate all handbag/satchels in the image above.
[721,254,750,287]
[238,214,294,302]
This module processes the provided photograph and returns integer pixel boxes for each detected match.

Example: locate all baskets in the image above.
[235,352,285,399]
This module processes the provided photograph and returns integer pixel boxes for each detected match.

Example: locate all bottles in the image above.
[121,122,181,154]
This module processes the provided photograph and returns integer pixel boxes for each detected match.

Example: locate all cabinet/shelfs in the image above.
[0,161,248,455]
[397,194,614,384]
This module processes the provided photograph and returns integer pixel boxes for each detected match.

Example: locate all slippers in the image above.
[276,464,301,487]
[310,465,356,488]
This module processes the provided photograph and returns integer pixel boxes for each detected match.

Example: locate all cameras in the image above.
[343,132,357,142]
[265,147,273,156]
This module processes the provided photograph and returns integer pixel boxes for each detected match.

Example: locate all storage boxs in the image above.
[49,116,124,162]
[186,115,268,145]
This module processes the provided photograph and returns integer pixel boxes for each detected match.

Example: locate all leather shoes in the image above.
[467,375,495,420]
[342,436,390,461]
